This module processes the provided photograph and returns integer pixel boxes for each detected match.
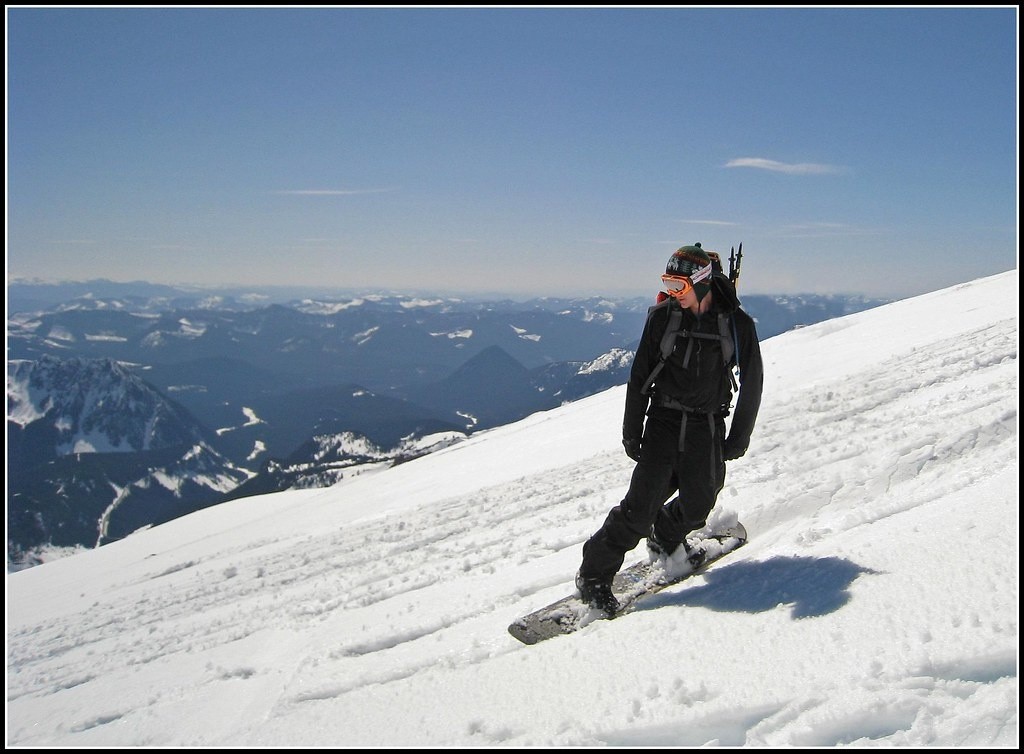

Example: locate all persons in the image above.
[575,243,763,617]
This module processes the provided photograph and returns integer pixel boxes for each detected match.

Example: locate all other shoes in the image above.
[575,570,619,617]
[646,525,706,567]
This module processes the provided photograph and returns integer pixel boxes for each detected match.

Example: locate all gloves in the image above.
[622,434,641,462]
[725,440,746,461]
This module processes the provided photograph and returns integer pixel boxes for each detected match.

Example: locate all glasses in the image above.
[662,274,694,296]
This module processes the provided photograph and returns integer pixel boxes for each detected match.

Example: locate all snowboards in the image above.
[507,522,748,646]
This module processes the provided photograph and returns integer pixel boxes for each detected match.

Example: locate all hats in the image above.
[666,242,713,330]
